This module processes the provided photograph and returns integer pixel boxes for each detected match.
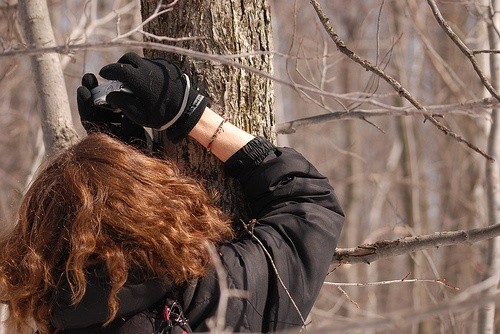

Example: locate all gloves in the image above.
[99,52,207,143]
[77,72,151,154]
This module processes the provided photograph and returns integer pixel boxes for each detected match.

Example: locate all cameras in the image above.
[92,79,134,113]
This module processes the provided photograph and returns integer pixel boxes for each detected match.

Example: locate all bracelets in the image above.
[205,115,231,151]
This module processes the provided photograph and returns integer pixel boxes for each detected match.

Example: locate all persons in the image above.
[0,52,345,334]
